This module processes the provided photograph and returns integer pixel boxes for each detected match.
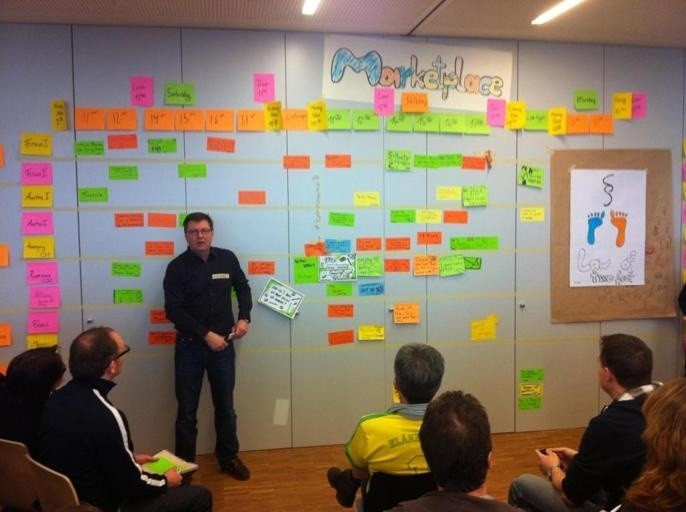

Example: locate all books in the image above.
[140,448,199,478]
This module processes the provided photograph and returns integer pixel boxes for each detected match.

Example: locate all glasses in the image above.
[187,229,210,236]
[114,344,131,362]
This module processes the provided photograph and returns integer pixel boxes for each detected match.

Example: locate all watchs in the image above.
[547,464,560,482]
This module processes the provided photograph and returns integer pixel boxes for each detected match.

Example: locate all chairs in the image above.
[357,471,439,512]
[0,438,79,512]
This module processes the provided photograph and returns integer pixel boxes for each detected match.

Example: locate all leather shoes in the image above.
[219,454,251,481]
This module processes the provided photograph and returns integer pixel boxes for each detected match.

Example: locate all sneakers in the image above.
[327,467,363,508]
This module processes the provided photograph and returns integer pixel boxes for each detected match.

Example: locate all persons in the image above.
[507,332,664,512]
[382,388,529,512]
[161,211,254,486]
[326,341,446,507]
[0,345,66,444]
[603,378,686,511]
[35,325,214,512]
